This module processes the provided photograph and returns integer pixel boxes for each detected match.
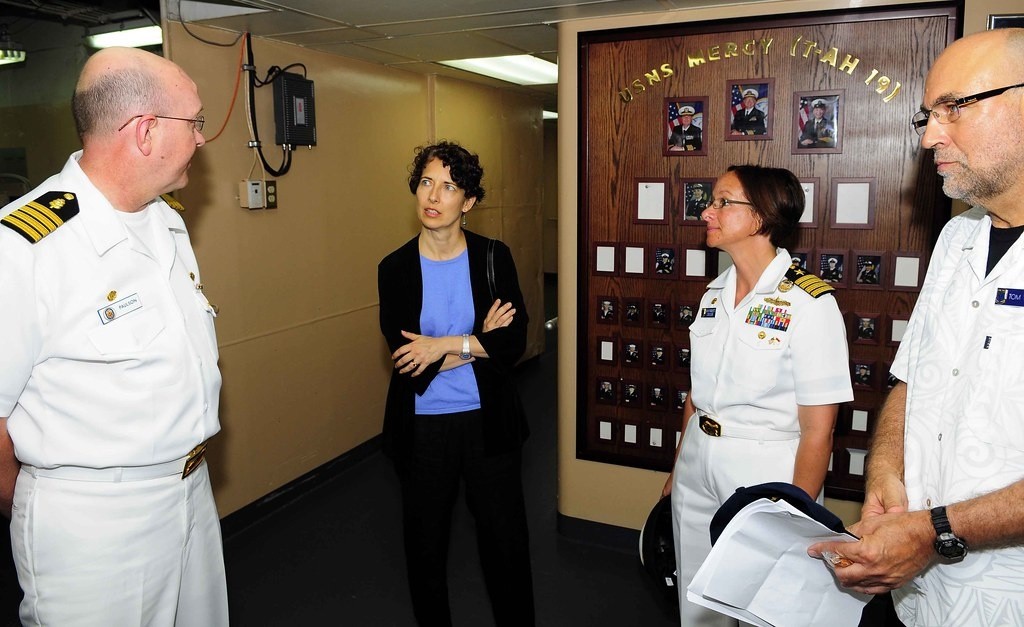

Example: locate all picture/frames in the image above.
[662,94,709,157]
[592,240,926,481]
[723,78,774,141]
[679,177,717,224]
[795,176,820,228]
[831,177,877,229]
[791,88,846,153]
[632,176,670,225]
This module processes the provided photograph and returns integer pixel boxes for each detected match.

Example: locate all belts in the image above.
[697,409,800,441]
[21,441,209,482]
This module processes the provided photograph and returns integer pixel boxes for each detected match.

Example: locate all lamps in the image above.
[82,9,162,51]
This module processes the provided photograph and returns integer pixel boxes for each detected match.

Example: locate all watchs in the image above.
[931,506,969,561]
[459,334,472,360]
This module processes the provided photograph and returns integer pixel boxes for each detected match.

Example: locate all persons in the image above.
[378,140,537,627]
[660,163,855,627]
[685,184,709,221]
[807,28,1024,627]
[600,301,692,411]
[668,106,702,151]
[797,98,835,148]
[0,46,229,627]
[655,253,672,274]
[791,256,877,384]
[730,89,766,135]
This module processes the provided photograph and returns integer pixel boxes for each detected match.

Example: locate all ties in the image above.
[745,111,749,118]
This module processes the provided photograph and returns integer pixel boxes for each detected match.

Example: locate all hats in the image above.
[865,261,873,265]
[710,483,862,548]
[860,365,868,370]
[742,89,759,99]
[811,99,828,108]
[692,184,703,189]
[828,258,838,263]
[662,253,669,258]
[792,257,801,262]
[862,318,871,322]
[678,106,695,115]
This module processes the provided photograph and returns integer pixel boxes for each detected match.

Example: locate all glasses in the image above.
[912,83,1024,135]
[119,114,204,131]
[706,198,752,208]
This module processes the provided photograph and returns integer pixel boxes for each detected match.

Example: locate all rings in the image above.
[412,360,418,367]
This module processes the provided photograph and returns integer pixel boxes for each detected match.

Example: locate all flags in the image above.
[668,102,683,140]
[755,84,768,128]
[818,95,839,147]
[691,101,703,142]
[731,85,745,125]
[798,97,810,141]
